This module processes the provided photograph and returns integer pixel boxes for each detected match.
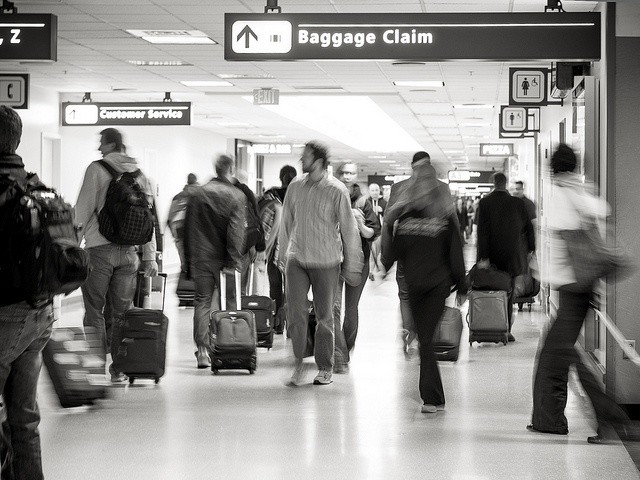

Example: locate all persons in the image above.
[332,160,379,375]
[72,128,159,381]
[476,172,524,343]
[0,103,56,479]
[512,180,539,310]
[379,164,467,415]
[168,173,201,276]
[365,182,389,281]
[259,166,299,334]
[184,154,247,368]
[100,140,167,354]
[383,151,430,358]
[525,142,636,443]
[277,143,364,388]
[211,171,264,335]
[454,193,480,236]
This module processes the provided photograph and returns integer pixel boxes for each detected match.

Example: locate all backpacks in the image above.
[94,160,157,245]
[166,192,191,240]
[0,172,91,308]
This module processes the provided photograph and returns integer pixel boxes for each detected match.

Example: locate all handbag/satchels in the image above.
[467,262,511,291]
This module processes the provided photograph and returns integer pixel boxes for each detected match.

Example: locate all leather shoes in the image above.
[526,424,569,435]
[588,434,602,443]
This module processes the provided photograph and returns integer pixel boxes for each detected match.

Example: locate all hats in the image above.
[550,141,578,165]
[411,151,431,168]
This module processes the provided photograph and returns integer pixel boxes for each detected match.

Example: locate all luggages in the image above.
[308,301,316,358]
[209,266,257,376]
[113,270,169,386]
[241,257,276,352]
[176,271,198,309]
[512,273,540,312]
[466,291,509,347]
[431,306,463,364]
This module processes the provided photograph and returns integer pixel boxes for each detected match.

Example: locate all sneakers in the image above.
[333,357,349,373]
[420,404,447,413]
[313,369,335,384]
[110,371,128,383]
[195,347,211,368]
[286,358,306,386]
